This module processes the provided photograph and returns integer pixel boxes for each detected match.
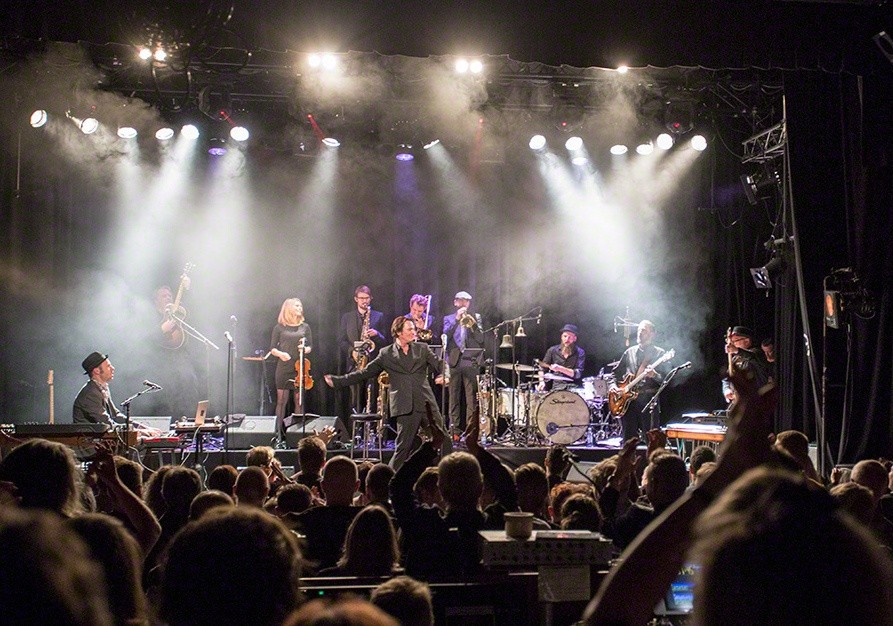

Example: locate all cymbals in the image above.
[494,359,540,372]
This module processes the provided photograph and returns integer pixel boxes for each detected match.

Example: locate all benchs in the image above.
[301,567,648,626]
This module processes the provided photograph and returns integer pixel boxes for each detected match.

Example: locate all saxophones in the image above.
[352,303,377,370]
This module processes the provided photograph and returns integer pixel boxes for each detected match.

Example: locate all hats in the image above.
[81,352,108,375]
[724,326,753,338]
[560,324,579,335]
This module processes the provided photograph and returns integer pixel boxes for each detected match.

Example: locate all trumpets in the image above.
[459,307,476,328]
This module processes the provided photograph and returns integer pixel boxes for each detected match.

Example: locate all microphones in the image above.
[440,333,447,349]
[302,336,307,347]
[142,380,162,390]
[230,316,238,323]
[224,331,232,343]
[614,318,618,334]
[673,361,693,372]
[537,309,543,324]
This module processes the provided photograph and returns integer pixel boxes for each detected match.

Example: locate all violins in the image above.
[292,338,315,390]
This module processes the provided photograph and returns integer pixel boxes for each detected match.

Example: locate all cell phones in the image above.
[653,559,703,616]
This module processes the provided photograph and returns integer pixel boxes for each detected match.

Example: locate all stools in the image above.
[351,414,383,465]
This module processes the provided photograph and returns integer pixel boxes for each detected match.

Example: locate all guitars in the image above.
[609,347,676,419]
[151,260,196,351]
[725,326,738,401]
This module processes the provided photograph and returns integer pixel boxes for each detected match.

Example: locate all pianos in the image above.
[141,427,188,449]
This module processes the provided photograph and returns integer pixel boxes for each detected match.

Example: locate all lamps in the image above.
[26,101,721,162]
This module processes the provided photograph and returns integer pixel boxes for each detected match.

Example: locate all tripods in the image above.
[477,305,543,448]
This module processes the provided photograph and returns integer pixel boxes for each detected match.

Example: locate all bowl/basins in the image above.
[504,511,534,538]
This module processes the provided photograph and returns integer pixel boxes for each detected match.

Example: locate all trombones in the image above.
[410,294,433,344]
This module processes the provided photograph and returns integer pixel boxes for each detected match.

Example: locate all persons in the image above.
[536,324,586,393]
[144,271,201,418]
[399,293,443,430]
[441,288,485,443]
[338,283,386,438]
[324,315,456,468]
[268,296,314,441]
[610,319,670,447]
[0,370,892,626]
[717,325,775,434]
[760,338,779,380]
[73,350,164,436]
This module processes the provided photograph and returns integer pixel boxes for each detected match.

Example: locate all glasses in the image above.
[730,337,749,345]
[357,297,370,300]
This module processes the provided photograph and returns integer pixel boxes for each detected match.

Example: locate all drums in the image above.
[496,370,617,446]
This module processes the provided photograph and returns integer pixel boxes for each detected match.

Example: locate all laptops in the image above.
[176,400,209,426]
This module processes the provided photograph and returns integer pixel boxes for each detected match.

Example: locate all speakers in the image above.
[224,416,278,450]
[288,415,349,450]
[565,461,600,485]
